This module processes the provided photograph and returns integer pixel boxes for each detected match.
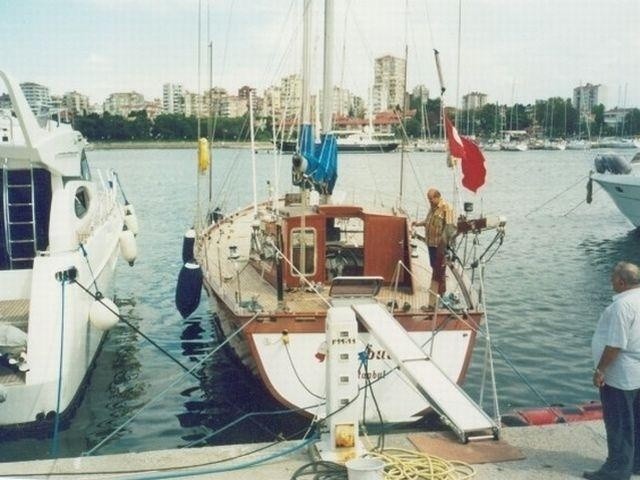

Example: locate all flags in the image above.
[444,116,487,194]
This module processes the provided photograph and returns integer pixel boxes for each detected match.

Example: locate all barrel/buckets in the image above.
[346,459,384,480]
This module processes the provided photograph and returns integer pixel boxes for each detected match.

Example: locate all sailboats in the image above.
[401,68,639,152]
[190,1,510,433]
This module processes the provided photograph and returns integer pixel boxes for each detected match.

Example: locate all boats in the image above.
[591,149,640,233]
[265,123,403,154]
[0,66,145,439]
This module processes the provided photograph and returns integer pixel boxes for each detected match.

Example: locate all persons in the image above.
[583,261,639,479]
[413,188,454,295]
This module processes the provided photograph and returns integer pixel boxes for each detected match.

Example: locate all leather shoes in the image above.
[582,459,640,480]
